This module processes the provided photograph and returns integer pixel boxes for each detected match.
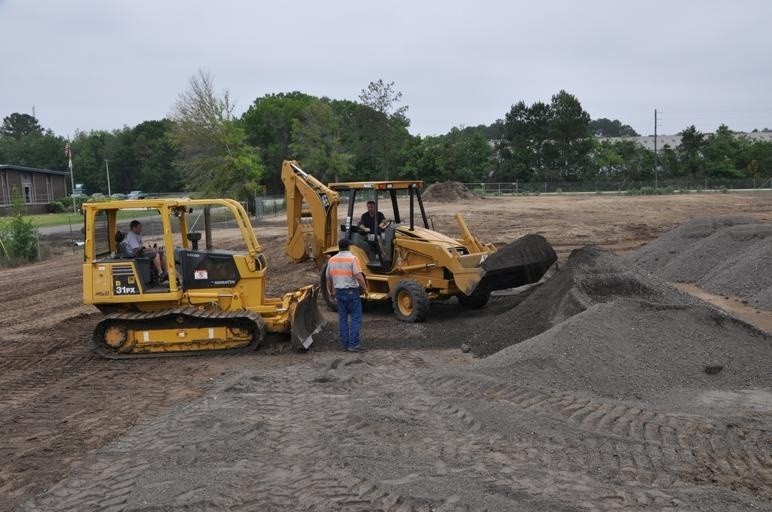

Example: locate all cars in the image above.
[72,189,145,202]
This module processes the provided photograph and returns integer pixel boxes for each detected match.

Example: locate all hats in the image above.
[338,239,354,248]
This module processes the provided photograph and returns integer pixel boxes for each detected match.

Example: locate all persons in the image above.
[121,219,166,279]
[326,238,370,353]
[359,199,386,259]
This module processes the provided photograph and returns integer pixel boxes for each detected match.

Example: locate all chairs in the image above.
[115,230,156,283]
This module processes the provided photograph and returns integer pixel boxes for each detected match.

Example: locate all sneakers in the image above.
[159,273,167,282]
[348,345,367,351]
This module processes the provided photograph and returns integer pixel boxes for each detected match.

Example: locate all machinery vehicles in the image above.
[281,159,558,323]
[80,198,328,361]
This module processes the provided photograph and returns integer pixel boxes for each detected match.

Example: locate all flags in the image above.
[68,151,73,168]
[63,141,69,157]
